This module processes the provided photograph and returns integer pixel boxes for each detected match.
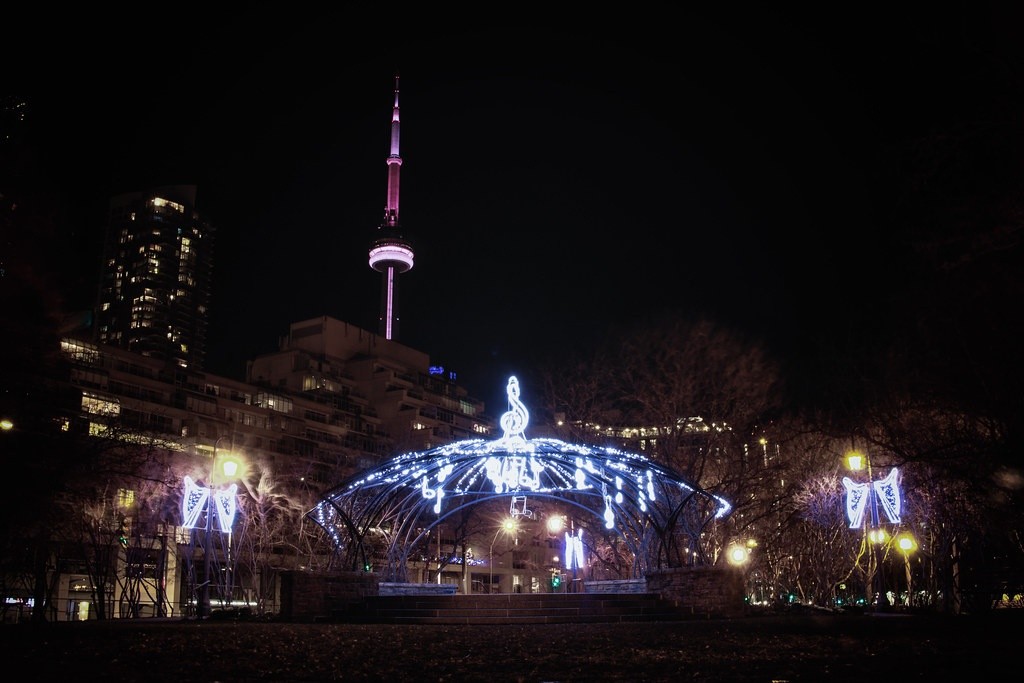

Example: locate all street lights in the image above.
[548,516,577,580]
[489,519,516,594]
[846,425,891,612]
[201,433,245,616]
